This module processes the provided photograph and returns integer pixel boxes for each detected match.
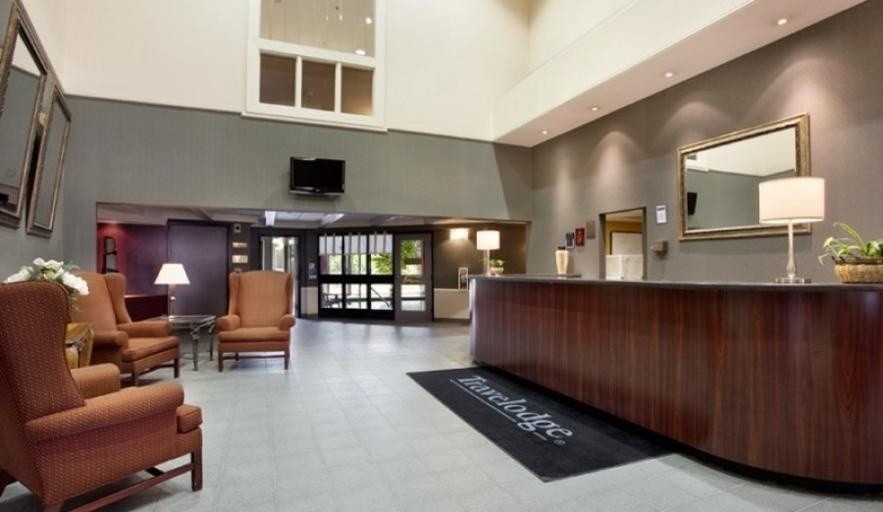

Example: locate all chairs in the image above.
[322,294,341,309]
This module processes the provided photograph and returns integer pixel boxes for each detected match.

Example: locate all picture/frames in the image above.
[575,228,584,245]
[565,232,575,248]
[586,220,596,239]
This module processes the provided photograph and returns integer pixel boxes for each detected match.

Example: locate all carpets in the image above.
[406,366,678,481]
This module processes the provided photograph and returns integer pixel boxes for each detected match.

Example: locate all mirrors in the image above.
[104,236,117,273]
[29,75,72,236]
[459,268,468,290]
[677,113,811,242]
[0,1,55,230]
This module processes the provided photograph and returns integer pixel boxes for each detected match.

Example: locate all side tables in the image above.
[148,315,216,371]
[65,322,94,368]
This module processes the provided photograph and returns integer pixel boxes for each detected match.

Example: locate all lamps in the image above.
[758,176,825,283]
[153,263,191,319]
[476,230,501,275]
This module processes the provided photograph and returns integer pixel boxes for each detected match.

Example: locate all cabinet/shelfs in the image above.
[466,274,883,485]
[434,289,469,319]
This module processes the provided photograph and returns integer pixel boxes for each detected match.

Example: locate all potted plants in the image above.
[818,221,883,283]
[489,259,505,275]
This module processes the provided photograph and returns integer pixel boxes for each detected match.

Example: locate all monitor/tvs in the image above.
[289,156,346,196]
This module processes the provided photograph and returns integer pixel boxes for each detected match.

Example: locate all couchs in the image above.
[214,270,295,372]
[103,273,174,324]
[71,272,181,387]
[0,280,203,512]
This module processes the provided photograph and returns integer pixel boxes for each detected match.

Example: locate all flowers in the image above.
[3,257,90,324]
